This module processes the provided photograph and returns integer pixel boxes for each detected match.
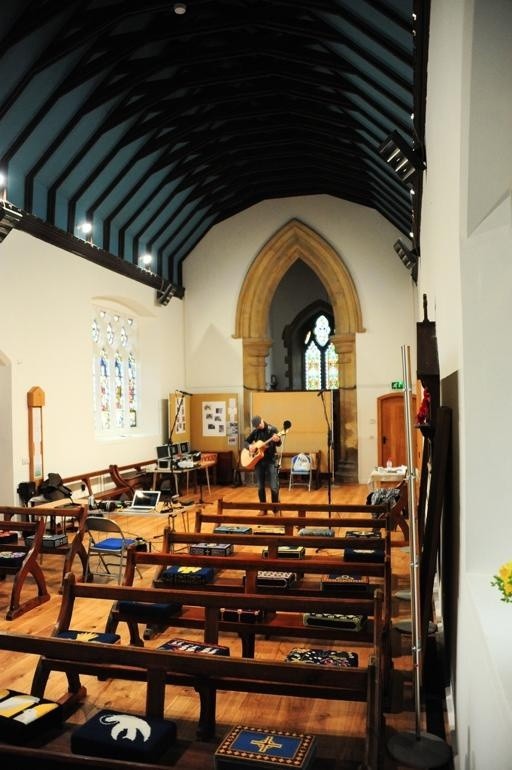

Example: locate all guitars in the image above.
[240,428,289,470]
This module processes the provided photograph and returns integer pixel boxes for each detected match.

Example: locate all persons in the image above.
[244,416,282,518]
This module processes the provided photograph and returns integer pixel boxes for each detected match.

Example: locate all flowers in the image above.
[491,562,512,604]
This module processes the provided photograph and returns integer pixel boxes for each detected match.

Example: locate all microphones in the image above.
[176,389,193,396]
[317,388,326,397]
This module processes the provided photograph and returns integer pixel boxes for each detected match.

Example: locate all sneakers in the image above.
[257,509,267,515]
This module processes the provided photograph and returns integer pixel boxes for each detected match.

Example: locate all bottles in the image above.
[387,458,392,470]
[193,452,202,467]
[88,496,94,507]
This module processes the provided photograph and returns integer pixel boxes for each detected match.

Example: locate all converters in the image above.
[82,484,85,490]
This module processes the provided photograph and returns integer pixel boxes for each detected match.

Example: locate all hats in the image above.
[252,415,261,428]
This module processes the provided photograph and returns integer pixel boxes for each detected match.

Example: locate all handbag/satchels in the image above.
[41,473,72,500]
[294,453,311,472]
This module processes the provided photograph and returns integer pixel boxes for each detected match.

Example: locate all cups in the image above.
[378,467,384,473]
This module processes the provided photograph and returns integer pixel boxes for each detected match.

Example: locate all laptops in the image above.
[123,491,161,513]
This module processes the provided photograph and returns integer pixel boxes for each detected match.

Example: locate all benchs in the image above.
[2,455,410,769]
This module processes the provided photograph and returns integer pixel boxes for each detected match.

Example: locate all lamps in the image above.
[390,240,417,272]
[157,279,175,306]
[376,130,426,183]
[0,202,25,246]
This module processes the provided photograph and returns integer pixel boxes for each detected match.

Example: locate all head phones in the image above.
[100,502,122,512]
